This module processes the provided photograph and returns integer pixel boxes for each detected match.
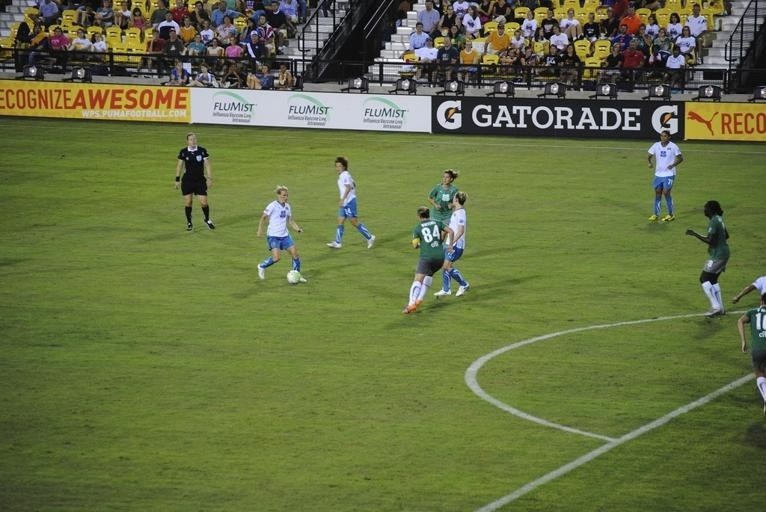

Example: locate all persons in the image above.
[403,207,454,314]
[175,133,215,231]
[326,157,376,249]
[409,0,708,85]
[732,275,766,304]
[737,292,766,416]
[427,170,458,242]
[685,201,730,317]
[433,192,469,297]
[257,185,307,282]
[648,130,683,221]
[15,0,308,90]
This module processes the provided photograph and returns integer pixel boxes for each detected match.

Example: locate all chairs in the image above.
[0,0,730,93]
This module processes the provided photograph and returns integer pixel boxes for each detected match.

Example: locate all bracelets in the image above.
[175,176,180,181]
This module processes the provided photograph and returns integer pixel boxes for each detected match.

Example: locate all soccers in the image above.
[287,271,300,284]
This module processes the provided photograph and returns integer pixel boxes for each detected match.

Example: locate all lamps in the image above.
[588,81,619,101]
[434,76,465,97]
[536,81,568,99]
[746,85,766,103]
[640,83,673,101]
[347,76,370,100]
[484,79,516,101]
[388,74,419,93]
[691,84,724,100]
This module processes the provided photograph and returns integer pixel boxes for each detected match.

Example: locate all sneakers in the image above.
[704,306,725,317]
[661,213,676,223]
[367,235,376,249]
[648,214,665,223]
[325,241,342,249]
[401,281,470,314]
[299,275,307,283]
[203,216,215,230]
[257,263,265,280]
[186,222,193,230]
